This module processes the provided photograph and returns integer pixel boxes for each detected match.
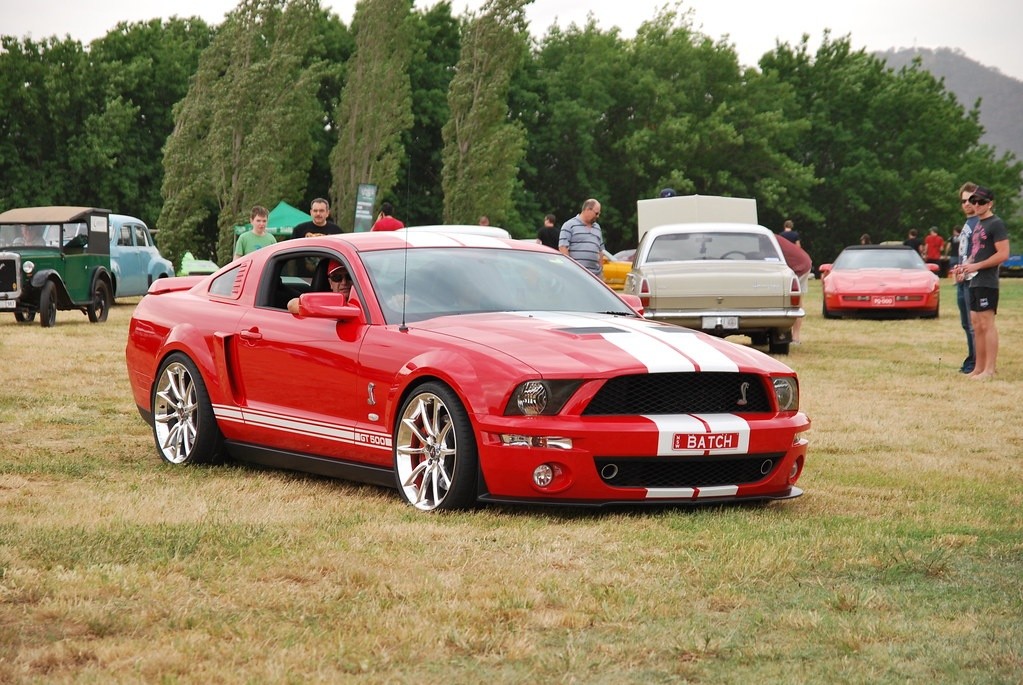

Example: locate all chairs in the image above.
[747,250,763,260]
[899,260,913,268]
[309,256,333,292]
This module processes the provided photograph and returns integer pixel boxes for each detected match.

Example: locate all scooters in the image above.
[0,206,117,328]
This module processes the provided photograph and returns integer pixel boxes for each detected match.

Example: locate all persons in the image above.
[291,198,345,238]
[369,202,404,232]
[660,188,676,198]
[903,226,945,278]
[781,220,801,246]
[480,216,490,226]
[535,214,560,251]
[944,224,962,286]
[948,181,1010,379]
[860,234,872,244]
[286,258,354,314]
[774,233,813,345]
[558,198,606,284]
[12,224,46,247]
[231,205,278,262]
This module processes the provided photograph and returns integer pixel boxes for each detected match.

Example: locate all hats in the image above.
[327,259,345,274]
[660,188,675,198]
[927,226,939,233]
[969,187,993,202]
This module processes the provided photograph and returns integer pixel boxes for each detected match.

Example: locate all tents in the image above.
[231,200,315,238]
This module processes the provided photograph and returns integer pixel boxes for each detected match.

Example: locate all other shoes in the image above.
[967,369,999,382]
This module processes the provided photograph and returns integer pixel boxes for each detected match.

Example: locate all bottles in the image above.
[962,255,972,280]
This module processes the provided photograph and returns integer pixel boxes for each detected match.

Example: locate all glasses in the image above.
[960,199,969,203]
[328,273,352,282]
[591,209,601,215]
[971,201,989,205]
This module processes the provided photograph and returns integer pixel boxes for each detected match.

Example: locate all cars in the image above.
[623,194,806,355]
[602,250,632,290]
[819,246,940,320]
[43,215,176,298]
[614,249,636,262]
[126,231,812,516]
